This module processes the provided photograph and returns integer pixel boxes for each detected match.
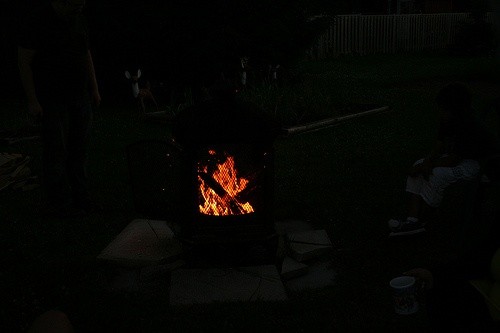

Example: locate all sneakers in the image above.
[386,217,431,239]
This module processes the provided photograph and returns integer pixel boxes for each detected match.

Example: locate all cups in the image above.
[390,276,418,315]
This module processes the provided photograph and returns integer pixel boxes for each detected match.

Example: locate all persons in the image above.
[385,83,488,265]
[418,238,497,333]
[29,1,105,218]
